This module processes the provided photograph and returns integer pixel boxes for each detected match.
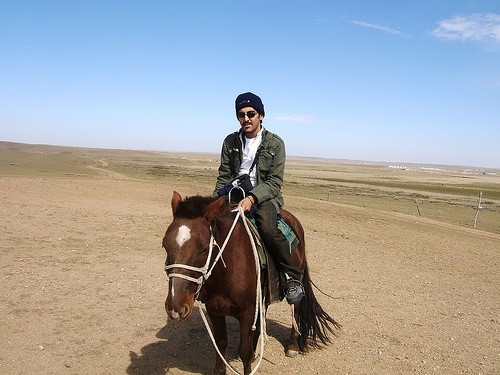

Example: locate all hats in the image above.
[235,92,264,117]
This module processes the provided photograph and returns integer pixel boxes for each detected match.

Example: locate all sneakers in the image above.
[285,278,303,305]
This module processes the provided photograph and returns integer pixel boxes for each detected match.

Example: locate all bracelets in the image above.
[246,196,255,205]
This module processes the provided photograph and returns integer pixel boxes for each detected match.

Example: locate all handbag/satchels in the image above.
[217,173,253,203]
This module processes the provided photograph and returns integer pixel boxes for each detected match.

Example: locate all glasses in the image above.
[237,111,258,118]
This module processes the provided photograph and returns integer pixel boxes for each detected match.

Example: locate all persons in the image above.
[215,92,304,305]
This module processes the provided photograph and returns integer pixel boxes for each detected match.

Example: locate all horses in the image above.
[162,191,342,375]
